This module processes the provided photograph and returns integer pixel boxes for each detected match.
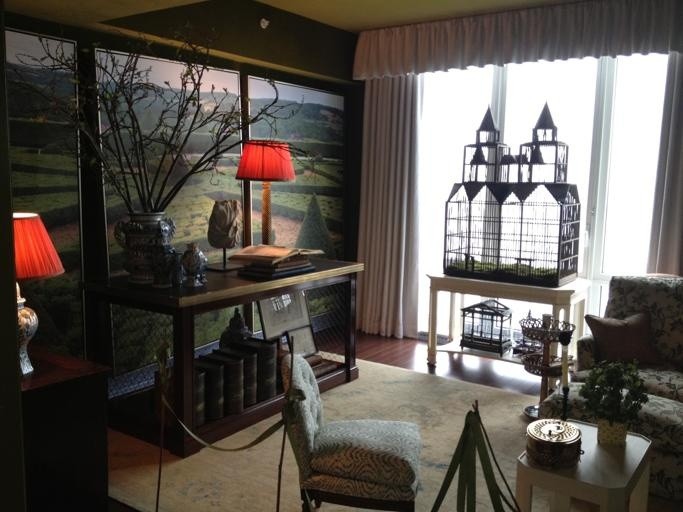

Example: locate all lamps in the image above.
[12,213,65,376]
[235,137,297,246]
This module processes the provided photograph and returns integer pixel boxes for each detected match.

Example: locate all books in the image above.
[226,241,326,283]
[305,353,338,379]
[151,334,278,432]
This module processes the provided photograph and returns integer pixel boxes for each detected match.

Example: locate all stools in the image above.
[538,381,683,504]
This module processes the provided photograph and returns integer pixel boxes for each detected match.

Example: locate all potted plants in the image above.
[582,359,649,446]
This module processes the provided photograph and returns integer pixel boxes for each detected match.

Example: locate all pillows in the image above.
[585,312,663,365]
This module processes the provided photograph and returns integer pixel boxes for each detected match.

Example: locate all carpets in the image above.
[108,346,572,512]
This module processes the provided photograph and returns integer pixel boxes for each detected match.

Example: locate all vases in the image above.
[114,212,175,283]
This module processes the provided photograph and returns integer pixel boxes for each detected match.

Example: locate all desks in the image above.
[425,272,590,391]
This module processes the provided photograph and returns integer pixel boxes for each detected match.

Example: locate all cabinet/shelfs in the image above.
[107,255,365,459]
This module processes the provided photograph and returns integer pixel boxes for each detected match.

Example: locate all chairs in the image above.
[282,352,422,512]
[540,274,683,400]
